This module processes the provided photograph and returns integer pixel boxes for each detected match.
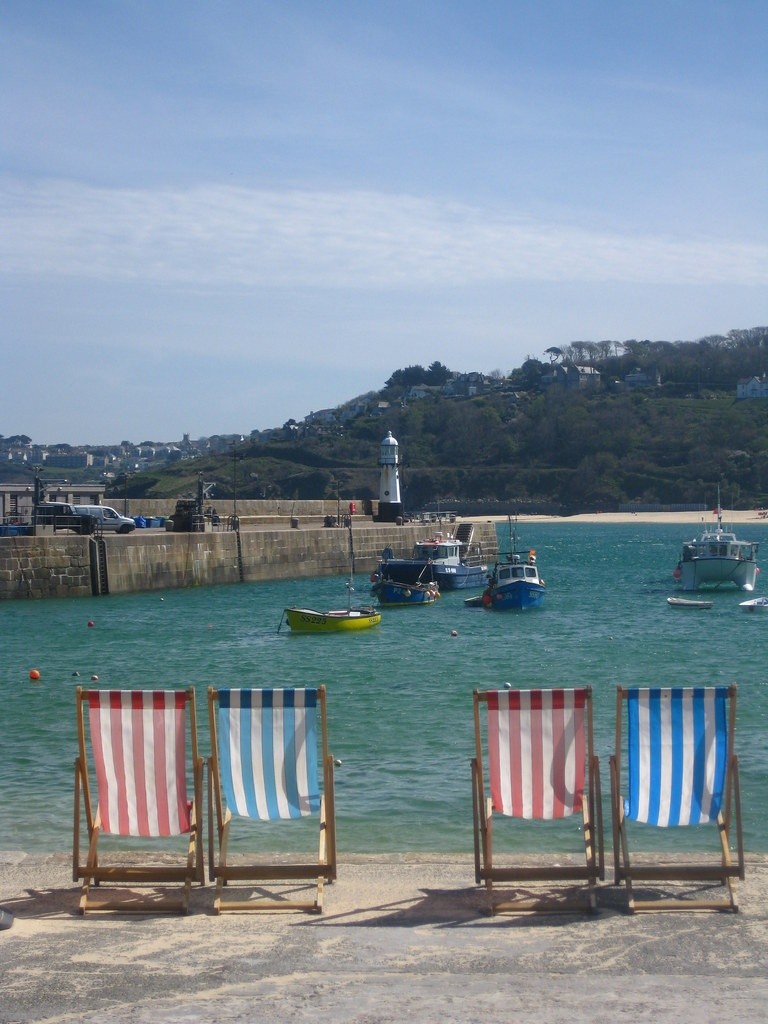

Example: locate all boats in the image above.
[464,596,483,608]
[371,580,440,607]
[673,481,760,590]
[285,554,382,634]
[378,492,489,590]
[667,598,713,609]
[486,498,549,608]
[739,597,768,612]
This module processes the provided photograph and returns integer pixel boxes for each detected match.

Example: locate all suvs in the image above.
[74,504,136,534]
[32,502,96,535]
